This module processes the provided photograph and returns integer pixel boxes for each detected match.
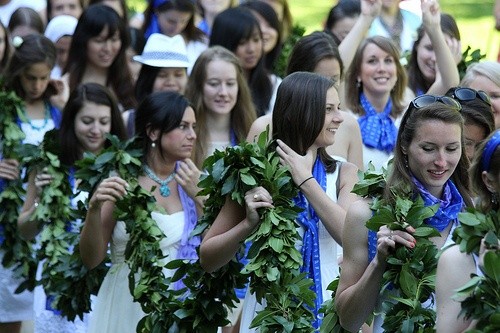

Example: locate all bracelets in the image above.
[298,176,314,188]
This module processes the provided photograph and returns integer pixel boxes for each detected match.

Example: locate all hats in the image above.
[133,32,192,68]
[46,15,78,46]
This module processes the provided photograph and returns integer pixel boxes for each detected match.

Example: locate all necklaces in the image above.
[19,102,50,130]
[142,165,176,197]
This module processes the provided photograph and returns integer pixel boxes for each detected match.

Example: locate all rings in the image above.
[253,194,259,201]
[390,230,394,234]
[388,235,393,239]
[36,175,41,180]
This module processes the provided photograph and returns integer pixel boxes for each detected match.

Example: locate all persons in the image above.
[434,128,500,332]
[0,34,61,333]
[334,95,473,333]
[17,82,126,333]
[0,0,86,82]
[198,72,360,333]
[78,92,220,333]
[57,4,138,114]
[89,0,292,118]
[245,30,364,301]
[183,47,257,176]
[323,1,500,181]
[120,32,193,140]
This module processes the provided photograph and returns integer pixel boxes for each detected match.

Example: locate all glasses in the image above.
[405,96,462,118]
[451,87,492,106]
